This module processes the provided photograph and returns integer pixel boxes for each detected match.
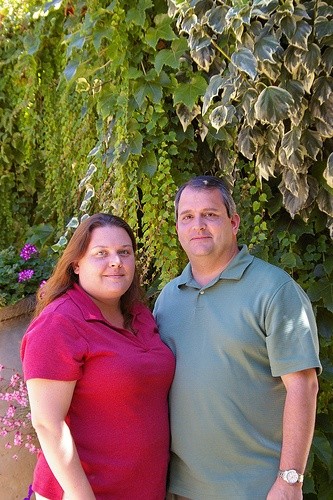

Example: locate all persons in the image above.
[152,175,324,500]
[18,213,177,500]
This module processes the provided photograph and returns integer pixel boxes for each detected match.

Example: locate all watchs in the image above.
[277,469,304,485]
